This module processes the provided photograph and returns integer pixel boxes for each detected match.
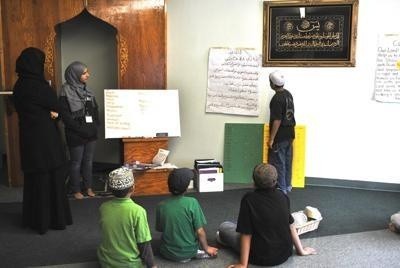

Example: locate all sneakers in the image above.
[193,250,216,258]
[216,231,230,246]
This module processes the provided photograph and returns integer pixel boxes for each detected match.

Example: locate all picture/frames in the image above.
[262,0,359,67]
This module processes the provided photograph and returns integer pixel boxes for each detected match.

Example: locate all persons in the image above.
[215,161,318,268]
[9,46,74,234]
[267,70,297,195]
[151,167,219,262]
[94,166,157,267]
[57,60,101,200]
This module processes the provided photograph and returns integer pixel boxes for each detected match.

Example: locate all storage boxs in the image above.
[194,173,224,193]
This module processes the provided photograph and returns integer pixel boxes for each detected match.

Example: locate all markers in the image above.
[123,136,154,139]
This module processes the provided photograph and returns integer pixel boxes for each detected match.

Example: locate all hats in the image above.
[168,168,195,195]
[108,166,135,191]
[269,72,286,87]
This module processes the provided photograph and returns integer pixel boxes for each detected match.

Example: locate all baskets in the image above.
[290,210,323,236]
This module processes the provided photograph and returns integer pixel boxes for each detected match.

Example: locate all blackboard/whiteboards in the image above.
[104,89,181,139]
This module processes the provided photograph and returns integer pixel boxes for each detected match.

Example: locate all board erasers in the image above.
[156,133,168,137]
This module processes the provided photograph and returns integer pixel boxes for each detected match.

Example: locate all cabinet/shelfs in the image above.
[122,137,171,196]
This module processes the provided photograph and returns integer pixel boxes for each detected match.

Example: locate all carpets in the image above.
[0,186,400,268]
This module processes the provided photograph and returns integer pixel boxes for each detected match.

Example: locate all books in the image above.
[194,159,223,173]
[144,148,170,169]
